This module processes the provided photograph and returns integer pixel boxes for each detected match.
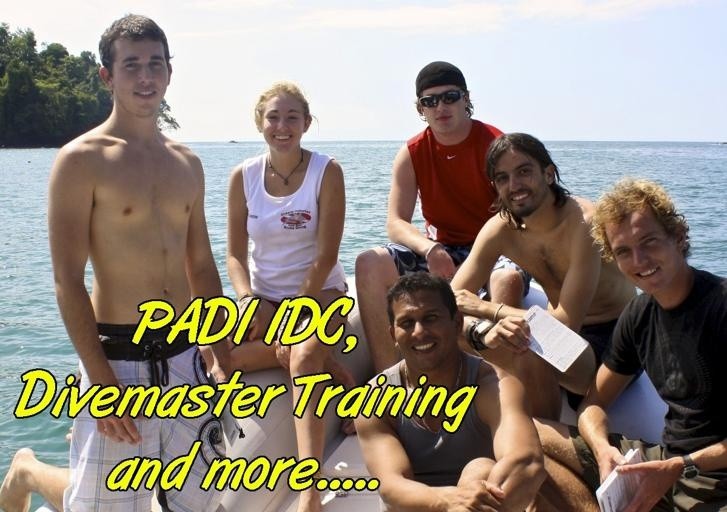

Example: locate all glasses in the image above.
[419,90,465,107]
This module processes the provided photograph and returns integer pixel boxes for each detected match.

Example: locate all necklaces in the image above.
[405,362,464,434]
[267,148,304,186]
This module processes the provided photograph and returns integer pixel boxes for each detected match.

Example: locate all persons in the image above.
[448,132,644,421]
[196,82,358,511]
[350,270,549,512]
[337,59,506,436]
[0,13,234,512]
[531,176,727,512]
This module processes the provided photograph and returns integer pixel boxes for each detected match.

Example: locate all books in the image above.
[594,446,646,512]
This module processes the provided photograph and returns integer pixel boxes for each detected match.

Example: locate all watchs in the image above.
[682,455,700,480]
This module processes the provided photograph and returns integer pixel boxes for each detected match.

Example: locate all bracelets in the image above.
[425,242,445,263]
[465,316,495,351]
[494,303,506,323]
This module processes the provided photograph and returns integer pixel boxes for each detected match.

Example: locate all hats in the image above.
[416,61,466,96]
